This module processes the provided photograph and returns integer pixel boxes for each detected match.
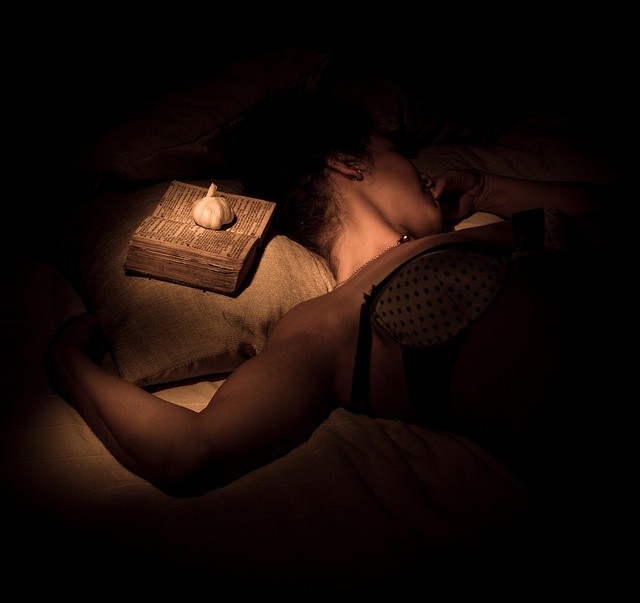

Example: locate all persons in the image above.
[37,82,639,500]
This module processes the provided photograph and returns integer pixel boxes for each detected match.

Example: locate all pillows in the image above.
[86,179,330,382]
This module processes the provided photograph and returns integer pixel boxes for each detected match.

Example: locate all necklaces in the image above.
[333,232,410,288]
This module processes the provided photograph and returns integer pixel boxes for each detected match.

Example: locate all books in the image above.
[122,177,277,298]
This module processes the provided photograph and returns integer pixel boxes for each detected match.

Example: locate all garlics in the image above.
[191,183,234,230]
